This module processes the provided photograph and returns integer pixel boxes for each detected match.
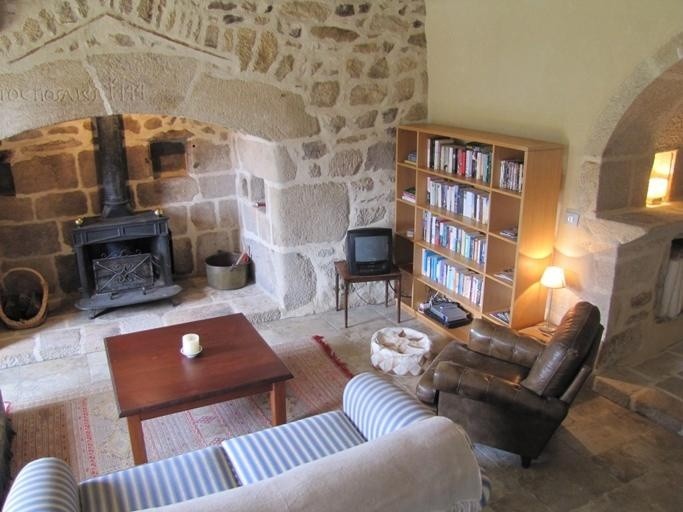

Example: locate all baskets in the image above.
[0,267,50,331]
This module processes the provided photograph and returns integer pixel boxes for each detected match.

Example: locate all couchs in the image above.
[1,372,491,512]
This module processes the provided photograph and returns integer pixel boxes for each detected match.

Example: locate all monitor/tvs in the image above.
[347,227,392,276]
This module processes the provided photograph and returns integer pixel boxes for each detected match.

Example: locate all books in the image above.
[403,137,524,326]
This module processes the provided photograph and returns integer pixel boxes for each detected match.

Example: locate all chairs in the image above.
[414,301,605,468]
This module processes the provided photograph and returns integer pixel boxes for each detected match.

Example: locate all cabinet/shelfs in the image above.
[392,121,569,346]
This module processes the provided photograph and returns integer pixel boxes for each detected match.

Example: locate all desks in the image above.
[335,260,402,329]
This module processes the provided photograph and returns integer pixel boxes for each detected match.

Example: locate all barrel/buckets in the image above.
[204,248,254,290]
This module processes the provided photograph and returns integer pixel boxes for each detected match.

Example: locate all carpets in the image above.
[5,334,354,500]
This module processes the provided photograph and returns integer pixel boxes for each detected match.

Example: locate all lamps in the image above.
[540,267,566,334]
